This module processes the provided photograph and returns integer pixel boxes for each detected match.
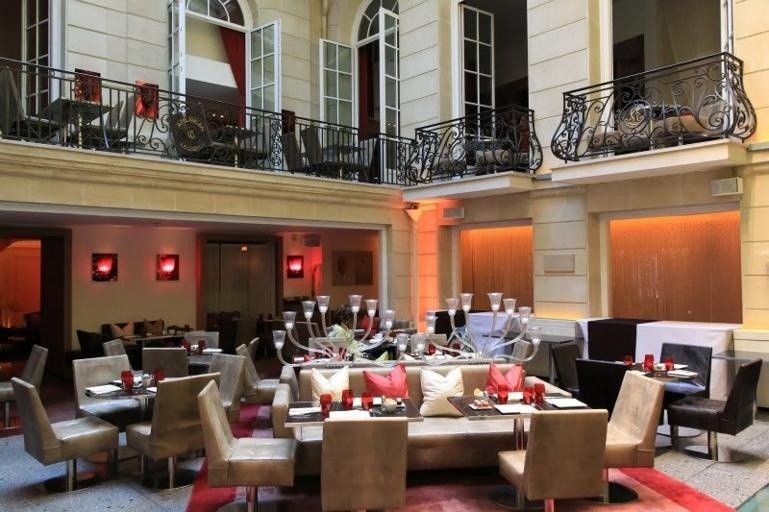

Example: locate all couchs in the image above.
[270,365,575,480]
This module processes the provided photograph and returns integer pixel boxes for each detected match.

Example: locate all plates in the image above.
[181,345,223,354]
[468,392,588,414]
[289,397,406,418]
[614,361,698,378]
[85,377,157,395]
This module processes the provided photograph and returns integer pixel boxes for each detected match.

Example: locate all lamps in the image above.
[157,254,179,280]
[287,256,304,279]
[92,253,118,281]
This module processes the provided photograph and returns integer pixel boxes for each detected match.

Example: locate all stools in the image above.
[587,104,725,156]
[433,116,528,180]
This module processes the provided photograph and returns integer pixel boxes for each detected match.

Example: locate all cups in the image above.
[121,368,165,393]
[314,347,347,359]
[428,344,460,356]
[320,389,373,417]
[497,383,545,406]
[624,354,674,372]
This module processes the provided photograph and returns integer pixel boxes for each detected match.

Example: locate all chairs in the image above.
[169,102,397,185]
[323,420,407,512]
[2,65,139,149]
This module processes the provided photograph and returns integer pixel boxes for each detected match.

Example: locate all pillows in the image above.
[363,362,412,400]
[311,369,351,401]
[420,369,467,416]
[486,364,524,392]
